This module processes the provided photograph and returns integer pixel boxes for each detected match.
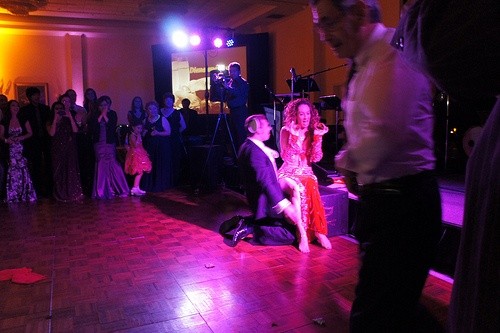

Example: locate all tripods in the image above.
[209,102,238,160]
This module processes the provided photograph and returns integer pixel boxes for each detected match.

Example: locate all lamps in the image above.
[225,31,237,48]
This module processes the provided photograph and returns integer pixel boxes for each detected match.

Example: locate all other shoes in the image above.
[130,186,146,196]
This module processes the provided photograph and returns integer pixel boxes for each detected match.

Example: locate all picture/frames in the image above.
[13,81,49,107]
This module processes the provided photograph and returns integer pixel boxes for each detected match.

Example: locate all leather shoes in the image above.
[233,219,248,246]
[219,216,245,235]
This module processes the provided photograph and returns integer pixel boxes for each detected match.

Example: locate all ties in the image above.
[345,62,356,100]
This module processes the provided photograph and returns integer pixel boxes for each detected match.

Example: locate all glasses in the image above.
[314,3,355,32]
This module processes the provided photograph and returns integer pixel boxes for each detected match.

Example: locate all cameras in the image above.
[57,110,66,116]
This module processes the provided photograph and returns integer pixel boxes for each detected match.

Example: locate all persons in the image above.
[403,1,500,333]
[0,62,331,253]
[313,0,444,333]
